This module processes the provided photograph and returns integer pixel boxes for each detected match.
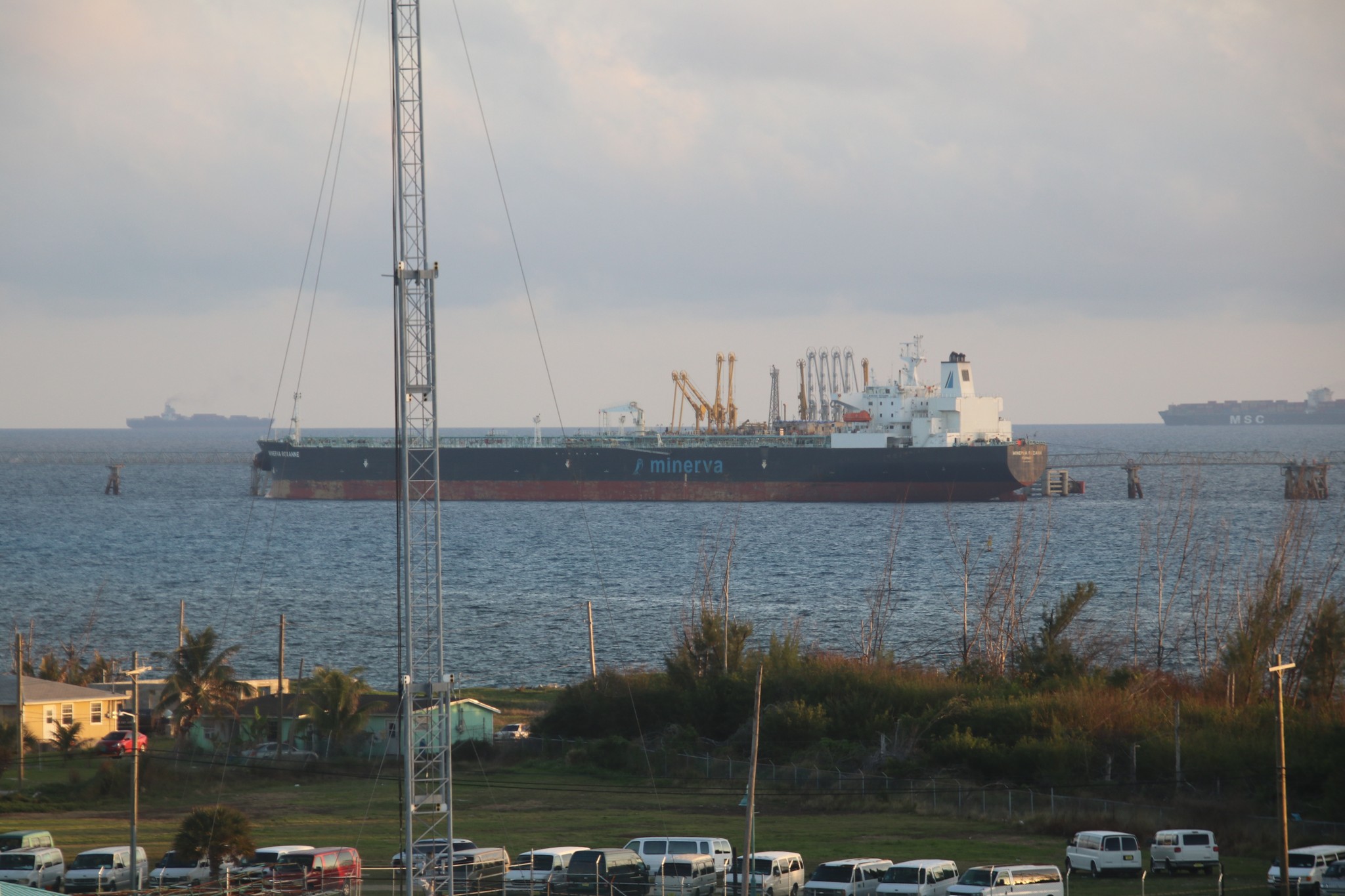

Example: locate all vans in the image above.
[623,837,805,896]
[946,864,1064,896]
[505,846,651,896]
[149,844,246,888]
[262,847,361,890]
[437,848,510,881]
[876,859,959,896]
[228,845,315,882]
[0,830,54,854]
[1148,829,1219,876]
[0,846,64,889]
[65,846,148,892]
[805,858,893,896]
[1267,845,1345,896]
[1065,830,1142,879]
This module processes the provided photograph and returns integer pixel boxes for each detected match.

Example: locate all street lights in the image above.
[118,710,138,889]
[739,783,757,896]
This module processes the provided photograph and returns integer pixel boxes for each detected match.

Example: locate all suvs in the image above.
[391,838,477,873]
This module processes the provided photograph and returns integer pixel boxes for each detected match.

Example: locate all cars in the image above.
[493,723,531,740]
[96,708,174,754]
[237,741,318,762]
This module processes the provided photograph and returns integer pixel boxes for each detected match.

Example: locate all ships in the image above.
[1158,400,1345,426]
[126,405,275,429]
[250,333,1047,501]
